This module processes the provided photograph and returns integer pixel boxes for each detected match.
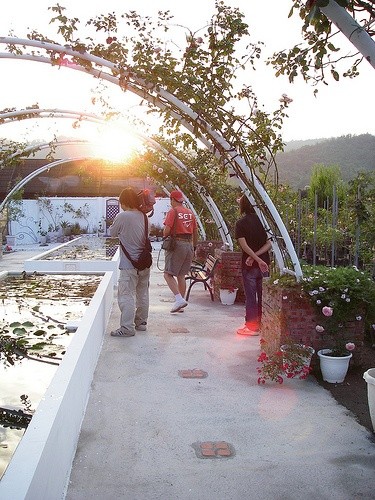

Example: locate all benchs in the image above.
[185,253,219,302]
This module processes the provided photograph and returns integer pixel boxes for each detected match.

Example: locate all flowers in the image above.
[256,334,315,387]
[312,294,364,355]
[213,260,240,292]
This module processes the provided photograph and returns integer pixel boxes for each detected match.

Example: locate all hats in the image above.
[169,189,185,202]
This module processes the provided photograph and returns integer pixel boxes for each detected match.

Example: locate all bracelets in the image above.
[194,247,196,251]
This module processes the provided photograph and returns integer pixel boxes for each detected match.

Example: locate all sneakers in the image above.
[237,326,261,336]
[170,299,189,313]
[111,326,136,337]
[135,321,147,331]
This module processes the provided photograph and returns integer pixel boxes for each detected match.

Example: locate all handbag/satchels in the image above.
[133,248,152,270]
[161,234,176,251]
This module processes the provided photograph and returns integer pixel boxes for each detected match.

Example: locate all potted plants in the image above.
[4,170,30,246]
[34,196,89,246]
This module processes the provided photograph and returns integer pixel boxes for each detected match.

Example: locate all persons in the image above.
[163,190,198,312]
[108,189,151,337]
[234,196,272,335]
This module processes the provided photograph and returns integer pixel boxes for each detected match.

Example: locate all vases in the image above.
[280,343,316,379]
[317,348,353,384]
[362,367,375,432]
[219,287,239,306]
